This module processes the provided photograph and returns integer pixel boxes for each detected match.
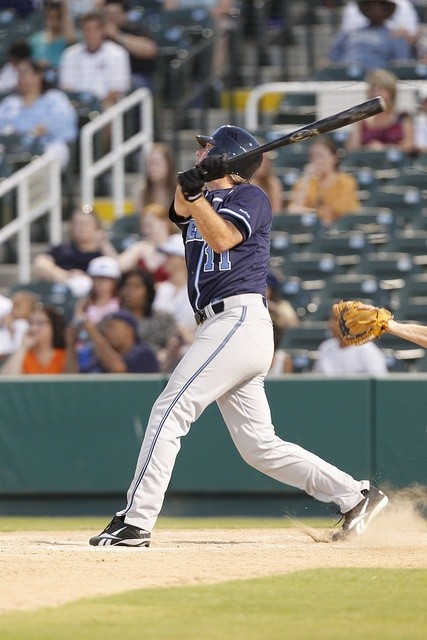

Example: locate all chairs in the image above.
[293,1,426,106]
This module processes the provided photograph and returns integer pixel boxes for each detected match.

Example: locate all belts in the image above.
[194,296,267,325]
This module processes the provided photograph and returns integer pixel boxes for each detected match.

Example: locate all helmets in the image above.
[197,125,263,180]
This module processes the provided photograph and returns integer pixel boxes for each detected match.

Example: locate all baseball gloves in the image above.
[333,300,394,345]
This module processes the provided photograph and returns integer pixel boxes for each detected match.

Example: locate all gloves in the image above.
[202,156,224,179]
[178,168,206,202]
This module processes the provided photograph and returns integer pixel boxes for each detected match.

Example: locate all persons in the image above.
[73,255,121,324]
[0,303,72,375]
[117,268,184,374]
[87,124,390,548]
[97,1,160,95]
[414,86,427,154]
[311,309,389,374]
[57,12,132,153]
[26,0,85,71]
[81,310,159,373]
[333,299,427,356]
[0,57,83,159]
[314,0,412,81]
[0,290,40,357]
[265,269,299,375]
[152,233,197,344]
[288,139,361,215]
[130,144,183,214]
[34,208,119,285]
[339,1,419,40]
[345,67,414,153]
[149,0,247,95]
[0,39,55,95]
[116,202,180,283]
[251,151,283,214]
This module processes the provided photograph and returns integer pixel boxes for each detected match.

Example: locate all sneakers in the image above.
[89,516,151,547]
[332,485,389,544]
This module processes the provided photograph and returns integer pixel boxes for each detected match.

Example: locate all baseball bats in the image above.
[177,95,389,186]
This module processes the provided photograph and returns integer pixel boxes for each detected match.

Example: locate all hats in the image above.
[106,309,141,345]
[156,233,188,257]
[88,256,121,280]
